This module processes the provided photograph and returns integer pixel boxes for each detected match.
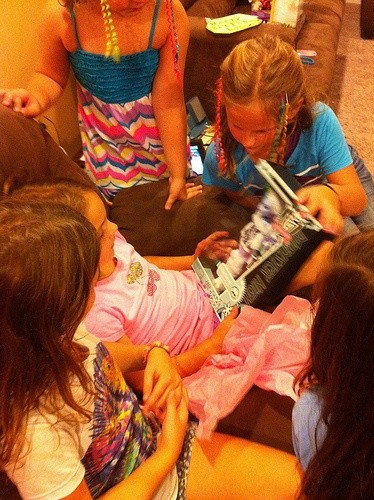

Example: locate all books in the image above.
[189,185,317,326]
[253,158,340,237]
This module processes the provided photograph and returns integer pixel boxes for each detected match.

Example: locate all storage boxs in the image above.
[190,157,338,324]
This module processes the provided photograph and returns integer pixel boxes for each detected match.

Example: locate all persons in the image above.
[199,32,374,246]
[1,177,374,500]
[0,0,206,212]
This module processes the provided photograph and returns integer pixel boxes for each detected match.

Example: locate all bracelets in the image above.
[185,351,196,374]
[141,338,172,365]
[173,353,192,376]
[179,353,191,373]
[320,180,343,206]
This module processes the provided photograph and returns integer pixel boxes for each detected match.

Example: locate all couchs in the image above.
[0,101,334,500]
[186,0,347,123]
